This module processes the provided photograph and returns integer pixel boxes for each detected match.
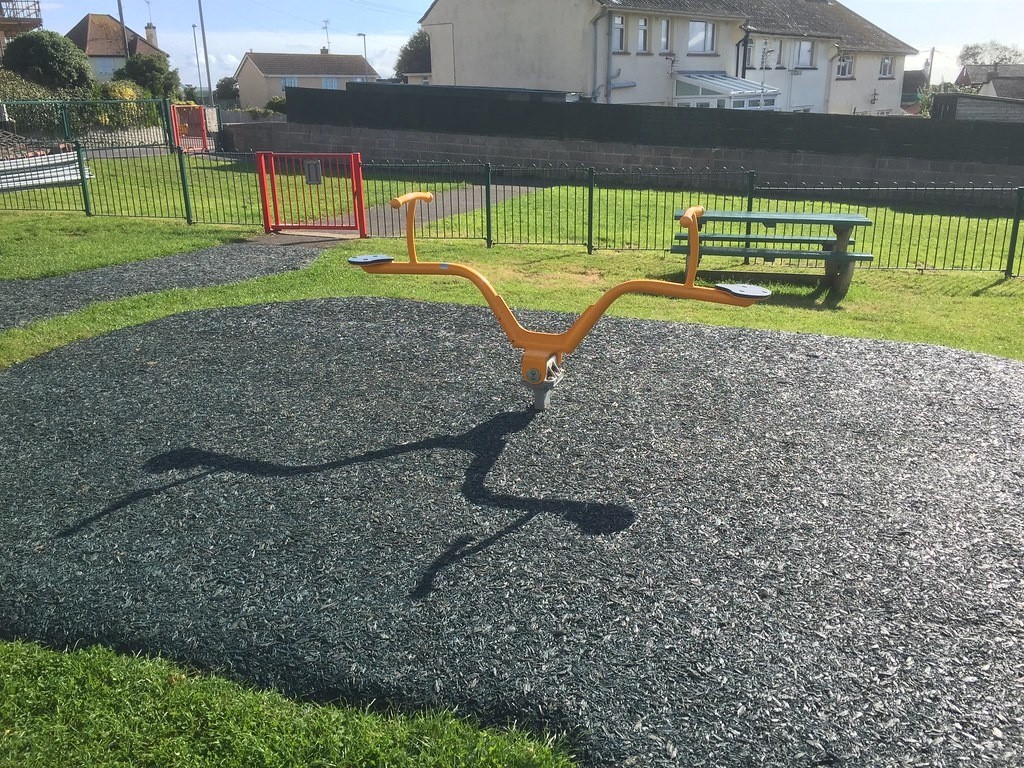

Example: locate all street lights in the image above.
[192,24,205,106]
[760,49,774,106]
[357,33,368,81]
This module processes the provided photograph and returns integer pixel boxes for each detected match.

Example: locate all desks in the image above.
[674,210,873,307]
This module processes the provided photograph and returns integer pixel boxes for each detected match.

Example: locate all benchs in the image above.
[675,233,856,245]
[670,245,875,261]
